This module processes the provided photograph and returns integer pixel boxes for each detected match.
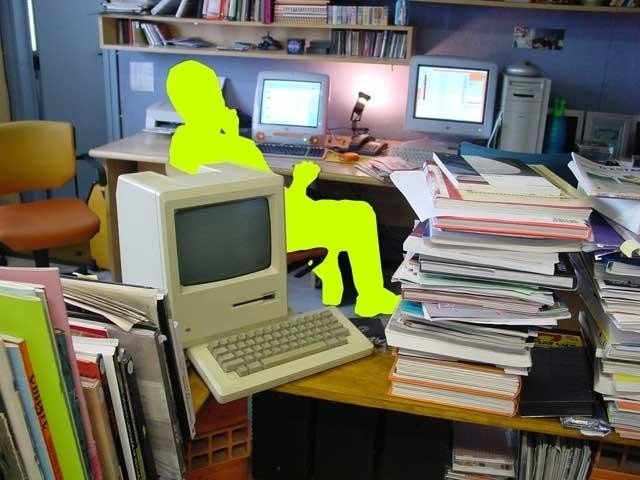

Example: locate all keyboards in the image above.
[255,142,328,160]
[186,304,376,407]
[388,145,434,163]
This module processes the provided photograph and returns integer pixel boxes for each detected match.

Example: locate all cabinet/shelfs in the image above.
[98,13,413,65]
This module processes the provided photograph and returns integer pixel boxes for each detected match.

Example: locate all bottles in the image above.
[395,0,408,25]
[544,98,567,152]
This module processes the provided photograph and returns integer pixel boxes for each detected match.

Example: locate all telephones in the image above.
[349,134,388,156]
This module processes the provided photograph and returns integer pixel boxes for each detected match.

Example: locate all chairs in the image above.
[0,121,100,267]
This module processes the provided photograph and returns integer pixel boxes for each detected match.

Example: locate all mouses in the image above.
[342,152,360,161]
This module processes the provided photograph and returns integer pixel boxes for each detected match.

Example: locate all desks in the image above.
[189,348,640,480]
[88,131,399,285]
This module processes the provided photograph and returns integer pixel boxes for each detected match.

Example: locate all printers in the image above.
[141,76,226,135]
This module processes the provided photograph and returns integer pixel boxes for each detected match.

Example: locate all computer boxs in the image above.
[497,72,552,153]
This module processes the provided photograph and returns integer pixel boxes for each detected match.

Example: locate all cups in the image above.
[287,38,305,54]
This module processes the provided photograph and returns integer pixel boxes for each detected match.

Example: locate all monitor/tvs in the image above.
[113,162,289,352]
[403,55,500,150]
[250,70,330,146]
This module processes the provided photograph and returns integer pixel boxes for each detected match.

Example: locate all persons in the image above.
[164,59,403,318]
